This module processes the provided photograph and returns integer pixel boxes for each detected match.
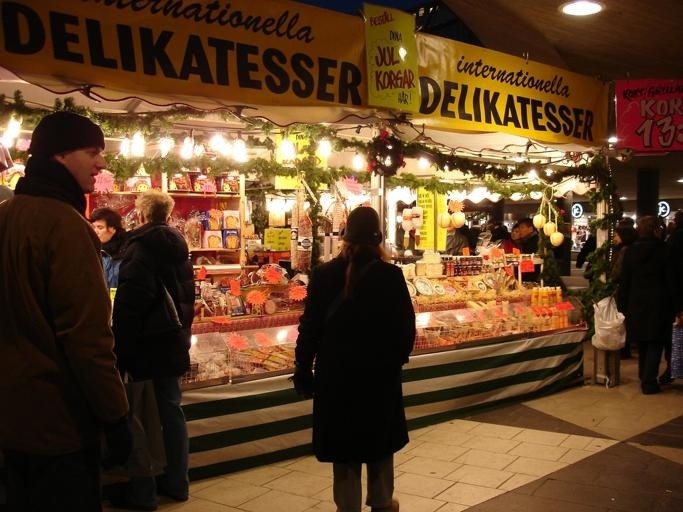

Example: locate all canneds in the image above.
[443,257,483,277]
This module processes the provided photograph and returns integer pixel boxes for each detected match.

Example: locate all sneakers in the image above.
[642,368,675,394]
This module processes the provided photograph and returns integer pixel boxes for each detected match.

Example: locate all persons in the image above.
[0,112,134,512]
[89,208,126,288]
[575,212,682,394]
[397,210,571,283]
[288,207,415,512]
[112,189,195,511]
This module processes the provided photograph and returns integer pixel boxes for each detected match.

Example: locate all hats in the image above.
[30,111,105,159]
[339,207,382,246]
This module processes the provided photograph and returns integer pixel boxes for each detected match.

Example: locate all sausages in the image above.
[291,203,299,270]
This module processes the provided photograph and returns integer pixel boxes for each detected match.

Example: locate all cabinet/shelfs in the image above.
[86,157,612,391]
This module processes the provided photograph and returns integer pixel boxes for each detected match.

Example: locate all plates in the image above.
[406,276,434,297]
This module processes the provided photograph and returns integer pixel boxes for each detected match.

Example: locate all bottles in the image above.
[465,216,472,229]
[530,286,569,330]
[440,257,454,276]
